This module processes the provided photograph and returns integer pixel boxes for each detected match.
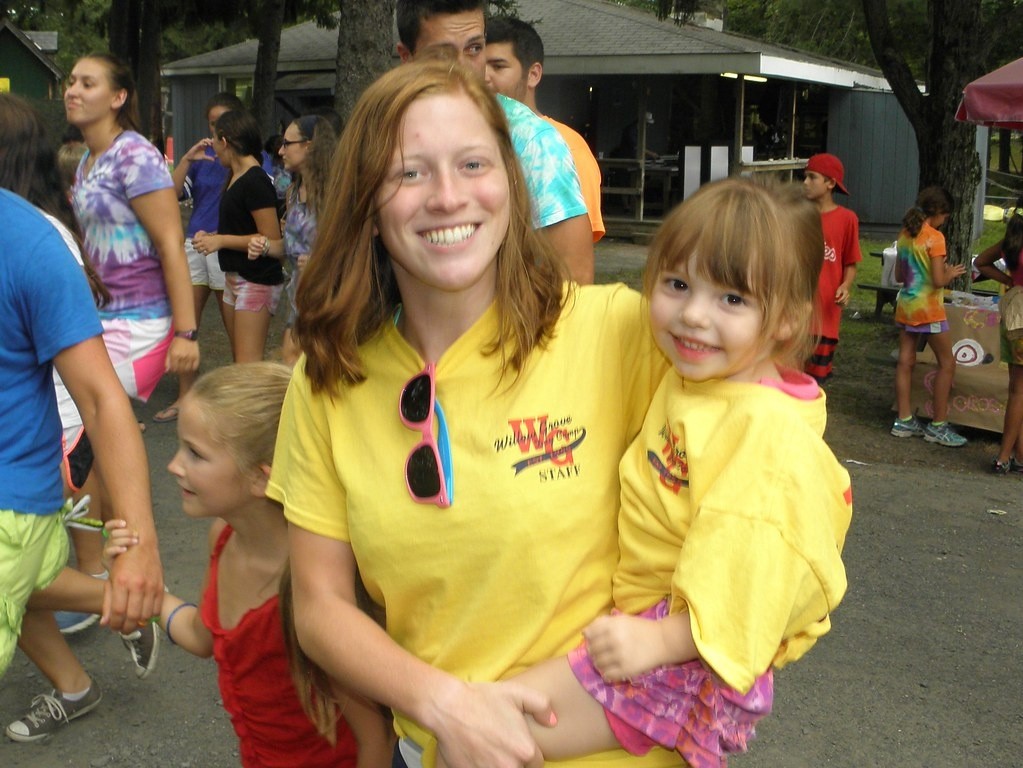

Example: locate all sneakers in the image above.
[5,673,102,743]
[924,422,967,446]
[118,584,169,679]
[890,414,925,437]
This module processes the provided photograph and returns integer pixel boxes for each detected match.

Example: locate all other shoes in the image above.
[992,459,1009,476]
[1010,458,1023,472]
[54,610,100,633]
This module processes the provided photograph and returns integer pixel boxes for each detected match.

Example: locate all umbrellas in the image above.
[954,57,1023,131]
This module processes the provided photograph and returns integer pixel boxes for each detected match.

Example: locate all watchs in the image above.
[175,330,197,340]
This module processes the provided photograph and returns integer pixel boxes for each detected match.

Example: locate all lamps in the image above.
[719,73,768,83]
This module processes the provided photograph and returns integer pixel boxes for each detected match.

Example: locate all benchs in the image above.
[857,283,1000,307]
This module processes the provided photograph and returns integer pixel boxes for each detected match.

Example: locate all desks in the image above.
[869,248,1006,316]
[627,165,678,215]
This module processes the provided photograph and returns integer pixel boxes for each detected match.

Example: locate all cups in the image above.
[645,159,652,168]
[598,152,604,160]
[656,158,663,165]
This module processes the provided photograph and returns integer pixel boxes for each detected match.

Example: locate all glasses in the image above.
[398,361,455,510]
[282,139,308,147]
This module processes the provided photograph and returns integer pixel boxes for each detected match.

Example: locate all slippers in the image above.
[151,407,179,421]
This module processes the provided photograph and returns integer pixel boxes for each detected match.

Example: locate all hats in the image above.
[805,154,850,197]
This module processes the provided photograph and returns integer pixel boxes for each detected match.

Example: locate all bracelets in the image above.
[166,602,198,645]
[262,240,269,257]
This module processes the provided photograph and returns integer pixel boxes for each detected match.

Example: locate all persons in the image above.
[248,115,338,372]
[798,152,863,383]
[973,192,1023,472]
[57,126,88,191]
[484,16,606,243]
[102,363,397,768]
[620,118,659,213]
[191,108,285,363]
[434,174,853,768]
[55,51,200,633]
[395,0,595,287]
[891,185,967,446]
[173,92,272,338]
[0,90,164,740]
[264,56,688,768]
